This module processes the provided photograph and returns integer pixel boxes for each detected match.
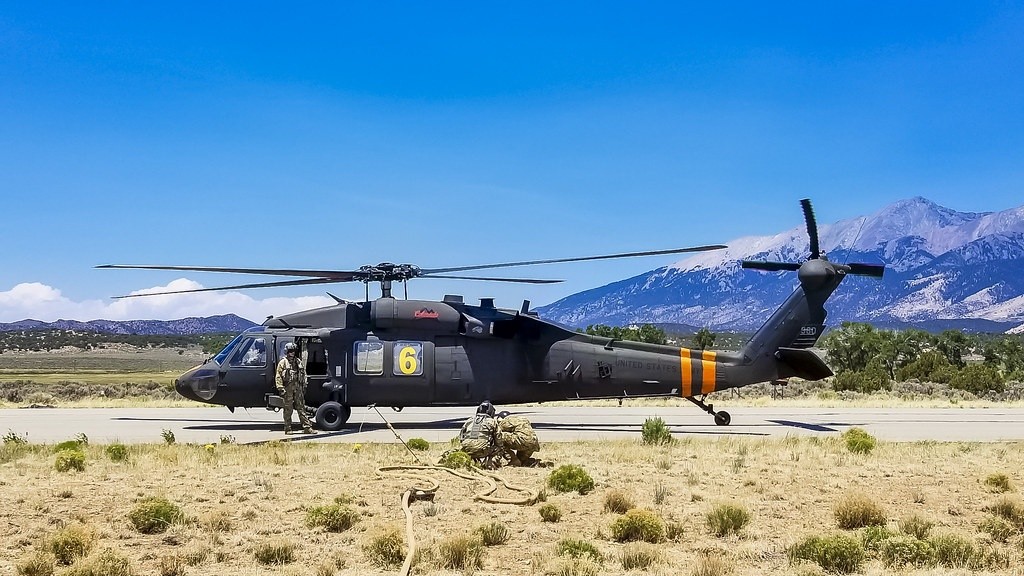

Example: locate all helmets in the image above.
[497,411,509,424]
[476,403,495,418]
[284,343,298,355]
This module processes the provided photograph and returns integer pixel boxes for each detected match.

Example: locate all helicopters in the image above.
[91,194,885,430]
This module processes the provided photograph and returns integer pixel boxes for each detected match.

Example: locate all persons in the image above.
[245,338,266,366]
[497,411,554,468]
[460,403,505,470]
[275,343,318,435]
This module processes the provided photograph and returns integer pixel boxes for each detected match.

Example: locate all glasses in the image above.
[289,349,296,353]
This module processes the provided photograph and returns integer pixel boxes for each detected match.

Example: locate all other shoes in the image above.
[287,431,293,435]
[508,458,521,466]
[530,458,538,468]
[304,428,317,434]
[483,462,498,469]
